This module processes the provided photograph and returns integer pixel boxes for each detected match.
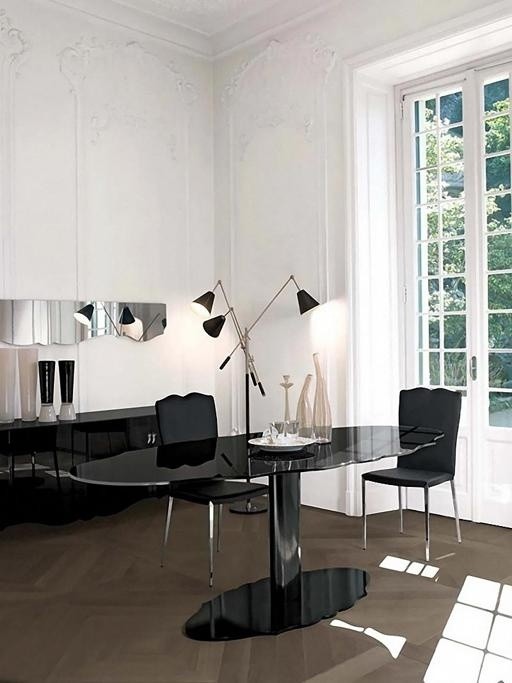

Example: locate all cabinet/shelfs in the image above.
[1,402,173,532]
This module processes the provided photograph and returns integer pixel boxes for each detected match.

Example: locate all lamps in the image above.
[190,272,324,516]
[71,299,165,342]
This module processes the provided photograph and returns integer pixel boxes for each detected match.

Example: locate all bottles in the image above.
[305,444,334,468]
[295,374,312,438]
[312,354,333,445]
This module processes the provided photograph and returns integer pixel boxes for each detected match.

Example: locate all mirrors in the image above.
[0,298,169,349]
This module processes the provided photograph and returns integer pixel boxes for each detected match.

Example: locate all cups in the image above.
[58,359,78,421]
[267,419,300,445]
[271,461,300,472]
[0,347,17,423]
[17,347,38,422]
[36,359,56,423]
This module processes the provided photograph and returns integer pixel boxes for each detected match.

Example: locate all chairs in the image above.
[154,390,272,590]
[358,385,465,562]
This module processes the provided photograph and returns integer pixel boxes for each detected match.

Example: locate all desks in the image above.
[67,424,447,644]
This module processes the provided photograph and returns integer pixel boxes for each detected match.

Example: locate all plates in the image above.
[248,438,315,452]
[249,450,315,462]
